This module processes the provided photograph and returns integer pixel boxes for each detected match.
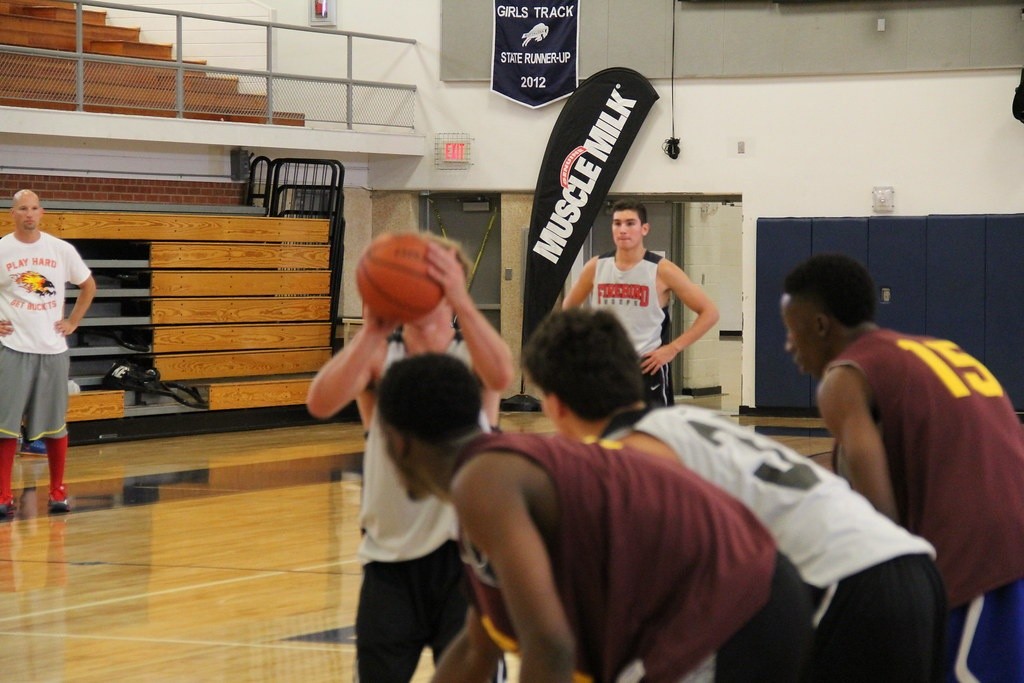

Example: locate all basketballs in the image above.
[354,230,448,326]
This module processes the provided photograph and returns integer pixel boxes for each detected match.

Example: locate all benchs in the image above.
[65,372,315,423]
[0,0,306,126]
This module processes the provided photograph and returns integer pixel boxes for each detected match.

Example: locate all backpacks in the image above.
[102,361,172,406]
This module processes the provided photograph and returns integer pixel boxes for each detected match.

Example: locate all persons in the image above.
[562,198,720,411]
[0,189,95,517]
[306,234,513,683]
[377,352,813,683]
[518,307,949,683]
[781,253,1024,683]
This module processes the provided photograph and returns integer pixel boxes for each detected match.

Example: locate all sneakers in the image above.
[0,494,16,521]
[48,484,69,512]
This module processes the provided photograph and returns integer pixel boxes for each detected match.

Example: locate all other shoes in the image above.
[22,438,47,453]
